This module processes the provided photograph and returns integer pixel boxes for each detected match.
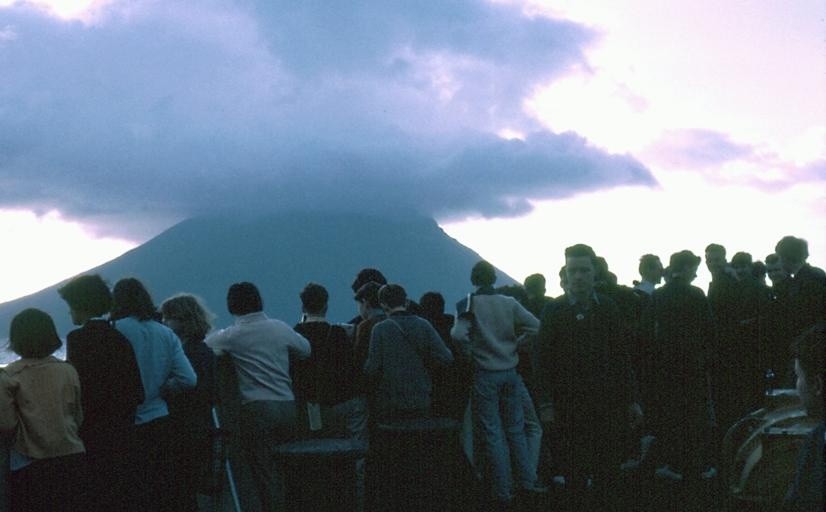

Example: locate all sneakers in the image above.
[519,481,550,494]
[654,461,720,482]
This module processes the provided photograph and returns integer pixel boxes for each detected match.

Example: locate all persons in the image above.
[159,292,218,510]
[203,281,312,510]
[108,279,197,512]
[58,273,146,511]
[347,235,825,512]
[0,309,88,511]
[288,282,346,512]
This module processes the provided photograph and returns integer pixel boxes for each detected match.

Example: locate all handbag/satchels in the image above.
[138,412,191,469]
[193,427,234,498]
[422,354,452,377]
